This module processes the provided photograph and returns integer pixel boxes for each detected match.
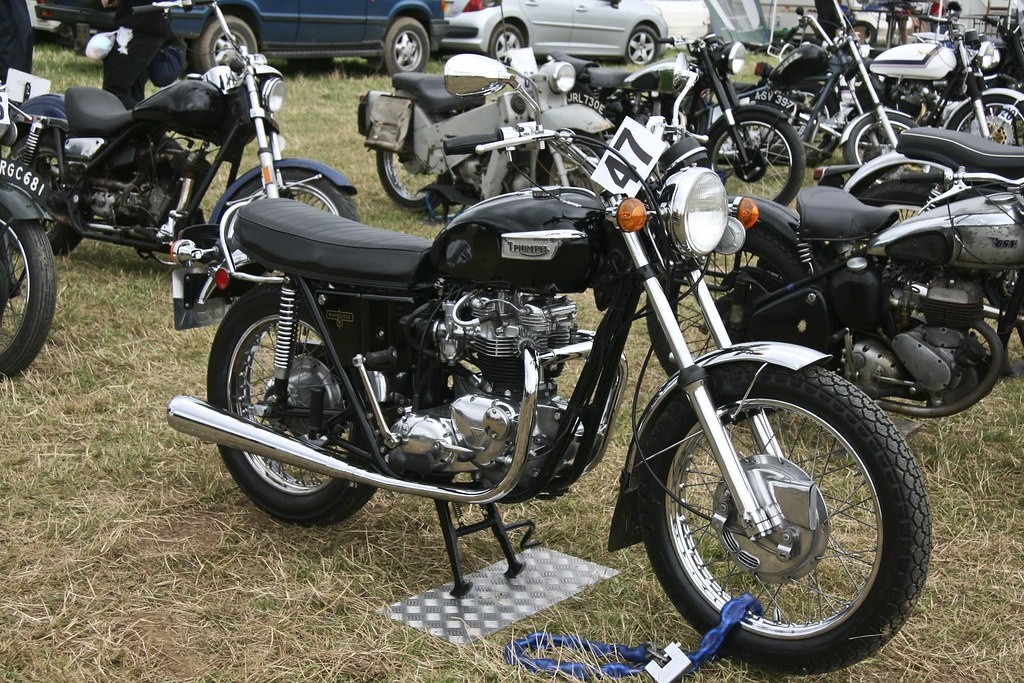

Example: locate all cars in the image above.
[430,1,713,67]
[31,0,451,75]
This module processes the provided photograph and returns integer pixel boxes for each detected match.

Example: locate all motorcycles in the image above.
[0,1,1023,674]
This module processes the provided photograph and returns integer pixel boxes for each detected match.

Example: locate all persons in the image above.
[101,0,174,110]
[885,13,909,48]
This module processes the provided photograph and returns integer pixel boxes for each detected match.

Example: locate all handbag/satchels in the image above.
[145,46,187,87]
[84,27,133,64]
